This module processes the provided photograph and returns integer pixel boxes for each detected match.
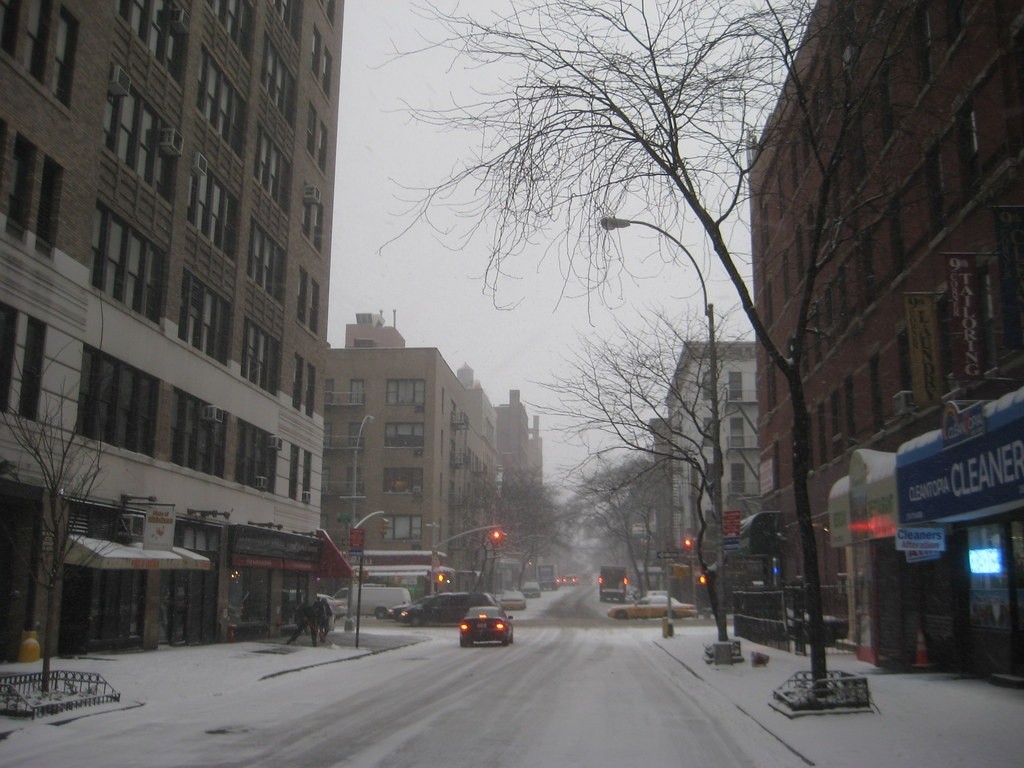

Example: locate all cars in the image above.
[498,591,526,610]
[315,594,348,619]
[459,606,513,648]
[388,591,500,627]
[521,582,540,598]
[607,590,697,619]
[556,574,576,586]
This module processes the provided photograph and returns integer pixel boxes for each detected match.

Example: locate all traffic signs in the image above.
[656,552,679,559]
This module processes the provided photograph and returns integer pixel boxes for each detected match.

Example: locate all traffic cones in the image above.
[912,629,934,667]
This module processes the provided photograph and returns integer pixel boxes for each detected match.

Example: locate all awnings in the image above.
[63,536,183,570]
[172,546,210,570]
[316,529,352,578]
[739,510,779,554]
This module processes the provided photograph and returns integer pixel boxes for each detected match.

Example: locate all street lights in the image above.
[603,217,726,640]
[346,414,375,629]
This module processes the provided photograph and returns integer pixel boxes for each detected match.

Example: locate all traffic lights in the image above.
[695,576,706,583]
[684,540,692,547]
[437,574,446,584]
[381,519,390,535]
[494,530,502,541]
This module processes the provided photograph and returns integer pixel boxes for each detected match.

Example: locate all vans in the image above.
[332,584,412,618]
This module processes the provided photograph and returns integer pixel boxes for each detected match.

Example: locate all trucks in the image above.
[538,565,557,590]
[600,566,627,601]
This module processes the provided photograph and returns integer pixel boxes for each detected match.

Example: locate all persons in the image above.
[287,598,332,647]
